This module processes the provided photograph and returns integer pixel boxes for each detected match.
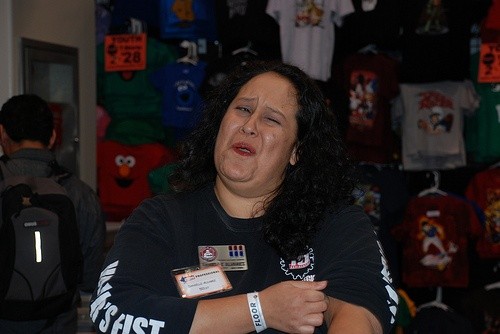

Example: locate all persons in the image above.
[87,57,399,334]
[0,94,106,334]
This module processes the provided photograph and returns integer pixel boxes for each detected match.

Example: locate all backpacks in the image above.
[0,153,81,322]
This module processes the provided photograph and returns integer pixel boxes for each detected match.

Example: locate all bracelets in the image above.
[247,291,267,334]
